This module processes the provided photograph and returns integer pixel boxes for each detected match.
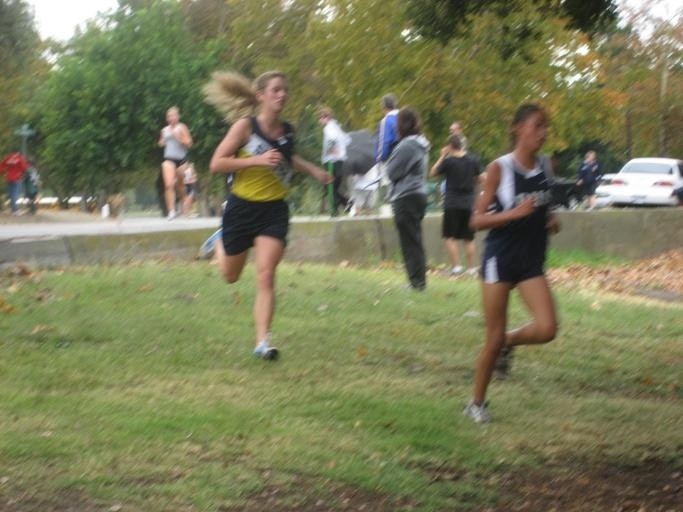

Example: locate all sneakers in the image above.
[198,227,225,261]
[343,196,355,213]
[405,283,427,293]
[494,346,513,381]
[447,264,480,282]
[462,398,493,429]
[253,338,280,362]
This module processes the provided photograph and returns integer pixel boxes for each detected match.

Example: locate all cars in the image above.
[611,154,683,209]
[551,169,587,211]
[596,173,616,206]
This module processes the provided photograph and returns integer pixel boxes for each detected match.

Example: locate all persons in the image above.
[459,101,563,427]
[20,158,42,215]
[382,105,435,292]
[155,103,194,221]
[0,147,31,217]
[194,67,336,363]
[314,104,360,221]
[427,135,481,282]
[374,92,402,166]
[575,148,604,208]
[180,159,197,220]
[447,121,468,152]
